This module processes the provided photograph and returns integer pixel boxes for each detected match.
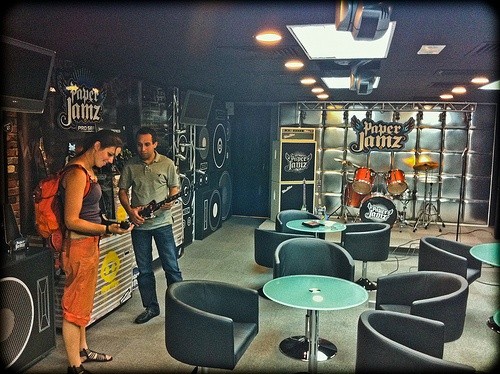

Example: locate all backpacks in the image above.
[33,164,90,239]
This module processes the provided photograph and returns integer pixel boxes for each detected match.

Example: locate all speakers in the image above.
[0,245,57,374]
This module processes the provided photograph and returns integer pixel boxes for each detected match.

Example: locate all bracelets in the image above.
[106,225,112,237]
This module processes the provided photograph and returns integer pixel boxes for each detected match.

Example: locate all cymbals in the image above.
[333,157,360,169]
[412,161,439,172]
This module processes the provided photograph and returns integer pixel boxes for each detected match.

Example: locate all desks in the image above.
[263,275,368,362]
[285,219,346,239]
[48,230,133,331]
[470,242,500,331]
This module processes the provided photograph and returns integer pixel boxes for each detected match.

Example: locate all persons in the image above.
[60,130,134,374]
[117,127,184,322]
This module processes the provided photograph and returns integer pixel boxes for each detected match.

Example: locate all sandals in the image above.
[67,364,92,374]
[79,349,113,363]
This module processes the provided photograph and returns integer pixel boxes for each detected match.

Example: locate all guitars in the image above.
[38,136,52,177]
[99,214,131,231]
[134,185,190,220]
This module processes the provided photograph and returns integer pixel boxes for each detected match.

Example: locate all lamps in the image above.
[334,0,393,42]
[348,64,380,95]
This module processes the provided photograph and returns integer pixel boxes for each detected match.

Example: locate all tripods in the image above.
[413,167,445,232]
[326,164,356,224]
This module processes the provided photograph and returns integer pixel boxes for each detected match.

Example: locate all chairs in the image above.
[165,210,476,374]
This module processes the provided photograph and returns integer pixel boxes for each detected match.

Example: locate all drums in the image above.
[351,166,376,196]
[358,192,398,229]
[395,188,416,200]
[343,181,371,209]
[382,168,408,196]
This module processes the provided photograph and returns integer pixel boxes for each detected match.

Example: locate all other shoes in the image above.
[135,307,160,324]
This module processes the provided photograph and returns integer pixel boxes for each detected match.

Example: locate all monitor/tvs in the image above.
[2,35,57,114]
[180,90,214,126]
